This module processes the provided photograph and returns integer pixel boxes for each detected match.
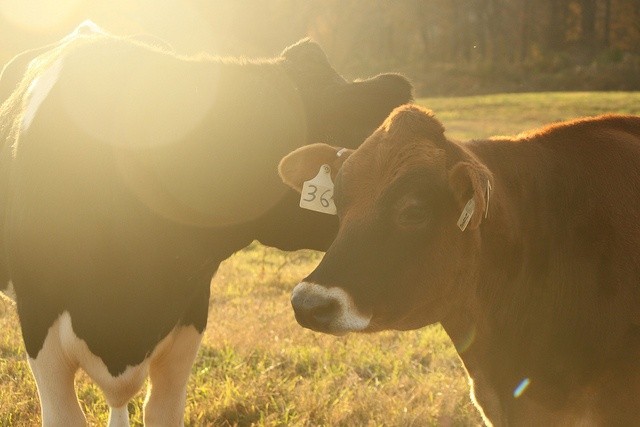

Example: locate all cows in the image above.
[1,22,415,427]
[278,111,640,426]
